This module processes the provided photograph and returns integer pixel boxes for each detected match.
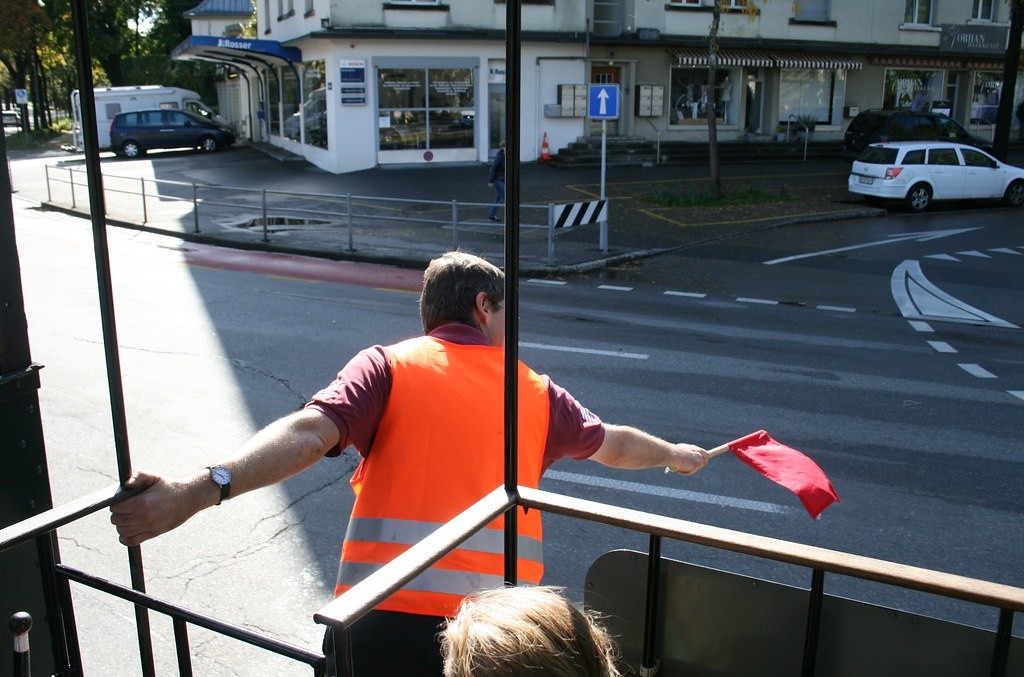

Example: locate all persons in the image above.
[899,84,912,107]
[435,581,635,677]
[111,250,711,677]
[487,141,506,221]
[721,81,732,102]
[972,95,979,109]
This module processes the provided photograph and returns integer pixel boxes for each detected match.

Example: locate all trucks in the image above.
[71,84,231,151]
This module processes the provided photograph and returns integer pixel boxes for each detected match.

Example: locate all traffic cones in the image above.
[541,132,553,160]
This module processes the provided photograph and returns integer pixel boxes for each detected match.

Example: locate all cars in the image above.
[2,111,22,127]
[847,141,1024,213]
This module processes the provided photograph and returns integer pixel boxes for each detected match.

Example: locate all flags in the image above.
[726,430,842,521]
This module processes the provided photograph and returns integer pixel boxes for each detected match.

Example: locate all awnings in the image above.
[170,35,302,73]
[763,48,863,71]
[666,48,773,68]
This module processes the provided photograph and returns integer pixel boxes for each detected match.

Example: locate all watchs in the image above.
[203,464,232,506]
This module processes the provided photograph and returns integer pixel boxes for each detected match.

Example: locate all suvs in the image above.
[844,107,994,156]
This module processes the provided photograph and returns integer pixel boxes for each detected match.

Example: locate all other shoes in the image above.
[488,214,501,222]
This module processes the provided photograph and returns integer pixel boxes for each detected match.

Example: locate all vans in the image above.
[110,110,235,159]
